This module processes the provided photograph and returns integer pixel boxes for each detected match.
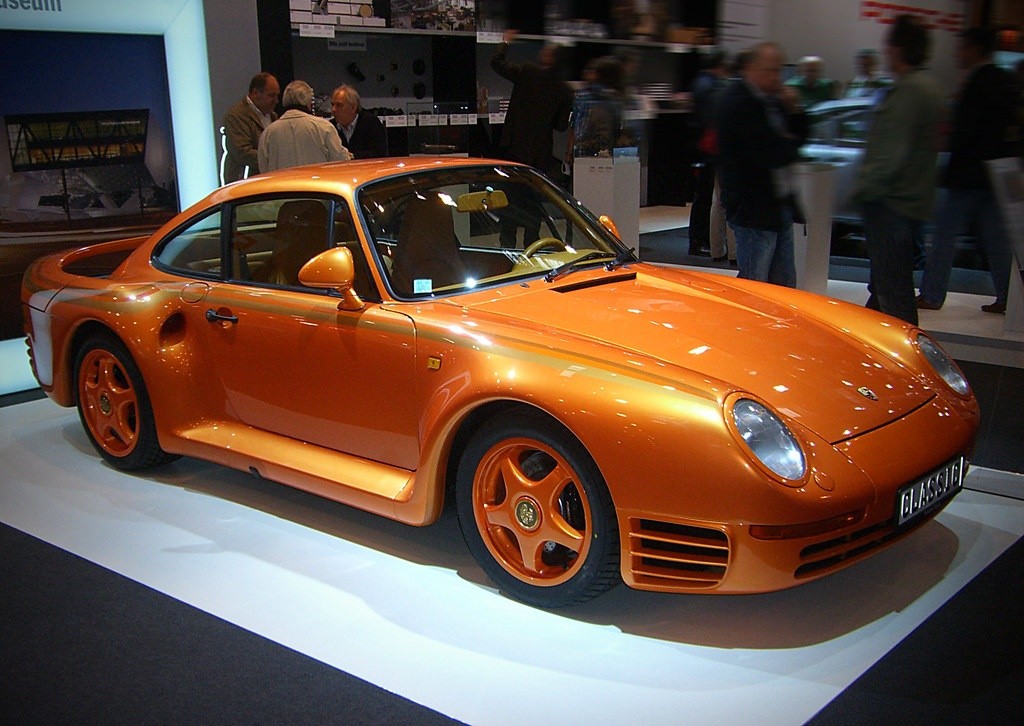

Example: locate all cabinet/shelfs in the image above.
[287,23,721,123]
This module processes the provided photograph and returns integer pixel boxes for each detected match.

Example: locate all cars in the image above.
[796,96,992,250]
[20,156,980,607]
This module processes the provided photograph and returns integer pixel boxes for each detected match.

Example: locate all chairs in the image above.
[390,192,474,297]
[267,200,334,291]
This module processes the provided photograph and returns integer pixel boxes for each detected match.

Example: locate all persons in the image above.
[785,55,837,110]
[842,48,894,138]
[257,80,351,174]
[490,29,574,250]
[687,42,807,289]
[914,22,1024,311]
[855,14,953,326]
[565,56,616,245]
[328,85,388,159]
[222,73,280,183]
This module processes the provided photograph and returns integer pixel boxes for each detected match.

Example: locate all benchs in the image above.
[187,239,387,302]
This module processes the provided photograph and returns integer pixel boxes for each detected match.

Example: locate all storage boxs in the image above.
[290,0,386,27]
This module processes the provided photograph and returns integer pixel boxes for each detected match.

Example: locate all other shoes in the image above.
[712,256,728,262]
[729,260,738,267]
[981,299,1006,314]
[915,294,942,310]
[688,244,711,256]
